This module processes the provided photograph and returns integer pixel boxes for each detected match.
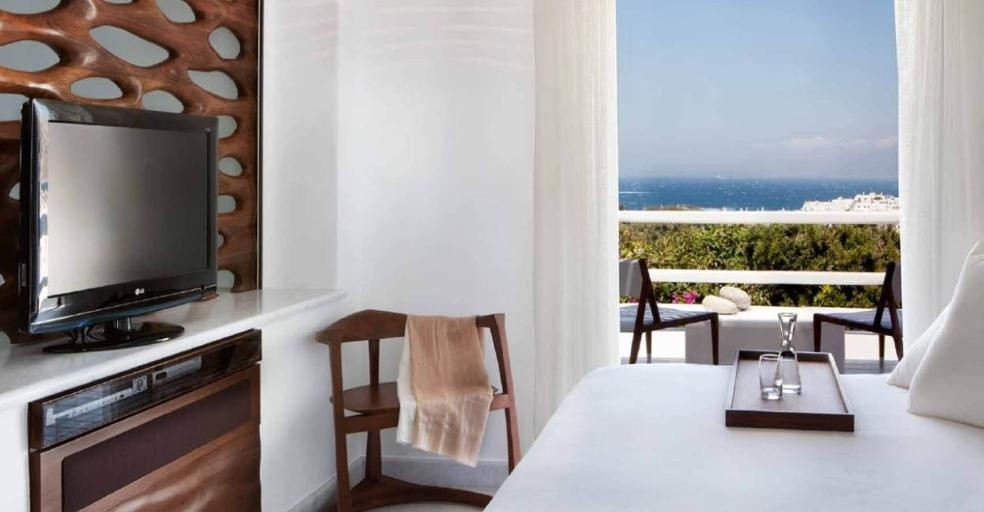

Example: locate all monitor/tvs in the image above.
[12,98,219,351]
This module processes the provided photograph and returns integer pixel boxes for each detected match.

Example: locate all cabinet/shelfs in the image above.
[30,360,263,512]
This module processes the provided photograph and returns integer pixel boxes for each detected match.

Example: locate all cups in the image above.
[759,353,783,402]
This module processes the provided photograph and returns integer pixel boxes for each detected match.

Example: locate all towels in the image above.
[888,240,984,428]
[619,259,718,364]
[396,314,496,469]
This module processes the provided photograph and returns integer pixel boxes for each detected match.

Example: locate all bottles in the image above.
[771,312,801,395]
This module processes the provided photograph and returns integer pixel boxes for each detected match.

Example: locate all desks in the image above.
[684,306,846,373]
[484,363,984,512]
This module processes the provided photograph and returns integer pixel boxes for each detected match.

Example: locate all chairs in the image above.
[315,309,521,512]
[813,260,903,374]
